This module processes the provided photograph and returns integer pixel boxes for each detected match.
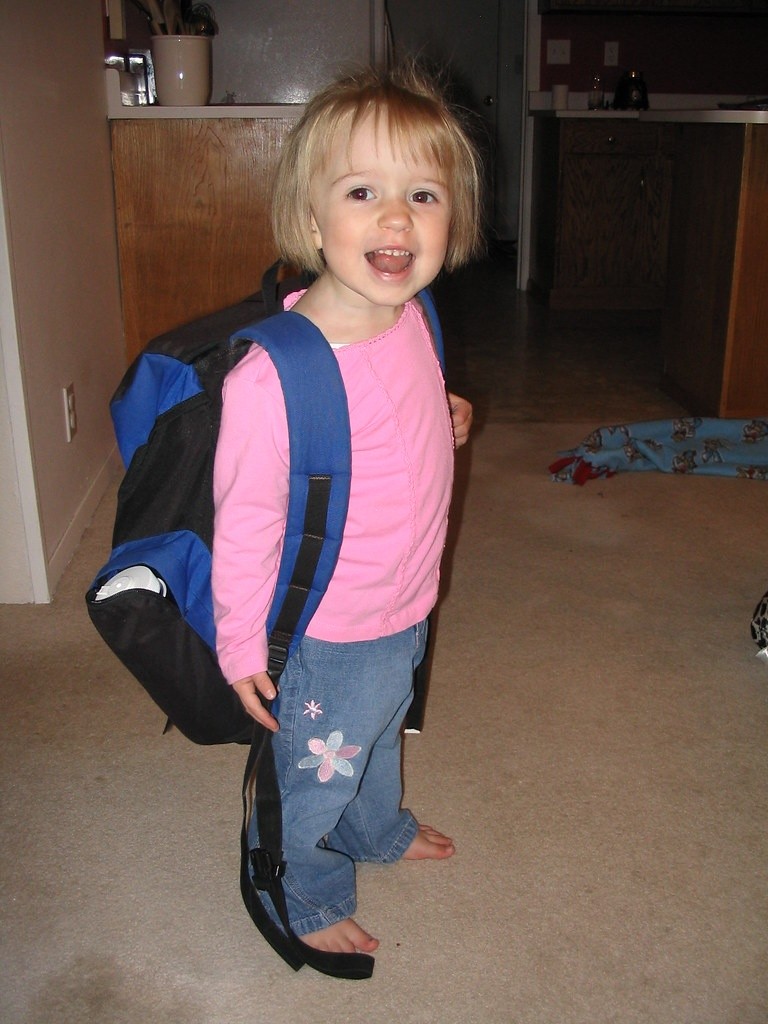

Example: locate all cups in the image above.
[588,79,604,110]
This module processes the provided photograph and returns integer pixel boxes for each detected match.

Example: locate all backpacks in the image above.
[85,260,451,748]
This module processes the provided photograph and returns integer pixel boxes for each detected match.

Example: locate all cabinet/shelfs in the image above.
[549,118,678,315]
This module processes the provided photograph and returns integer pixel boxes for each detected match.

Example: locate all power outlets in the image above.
[604,40,619,67]
[63,382,79,443]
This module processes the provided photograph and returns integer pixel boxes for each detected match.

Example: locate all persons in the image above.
[212,51,478,953]
